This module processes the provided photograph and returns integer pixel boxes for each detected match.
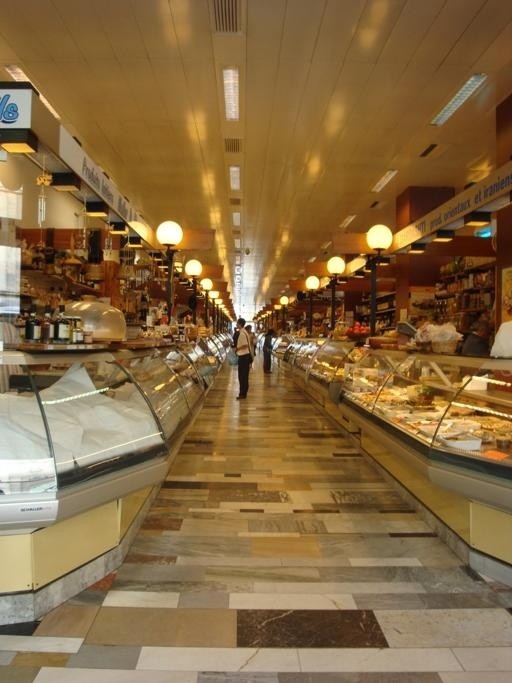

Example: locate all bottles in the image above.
[68,315,93,344]
[25,305,41,340]
[40,305,55,342]
[54,304,70,344]
[432,274,487,331]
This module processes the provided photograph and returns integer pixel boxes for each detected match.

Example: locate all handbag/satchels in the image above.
[267,346,272,353]
[249,353,253,363]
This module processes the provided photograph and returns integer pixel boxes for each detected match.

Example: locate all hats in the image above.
[463,309,484,330]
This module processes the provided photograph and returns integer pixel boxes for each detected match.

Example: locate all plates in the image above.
[374,394,511,450]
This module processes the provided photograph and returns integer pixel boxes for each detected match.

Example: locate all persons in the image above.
[262,328,275,373]
[458,319,491,357]
[231,317,253,400]
[232,324,258,368]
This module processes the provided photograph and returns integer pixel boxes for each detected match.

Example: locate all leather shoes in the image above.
[264,370,272,373]
[237,395,246,399]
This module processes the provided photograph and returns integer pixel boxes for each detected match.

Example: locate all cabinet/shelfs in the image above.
[358,292,396,329]
[435,262,499,340]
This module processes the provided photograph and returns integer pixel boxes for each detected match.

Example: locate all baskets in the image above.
[430,340,457,353]
